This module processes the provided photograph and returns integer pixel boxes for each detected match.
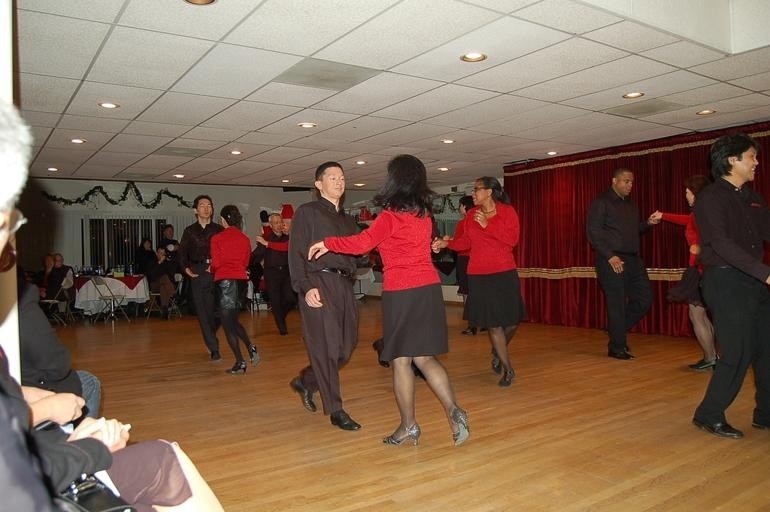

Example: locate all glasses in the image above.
[472,187,486,192]
[3,212,28,235]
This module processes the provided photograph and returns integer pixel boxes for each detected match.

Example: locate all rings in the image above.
[615,267,618,270]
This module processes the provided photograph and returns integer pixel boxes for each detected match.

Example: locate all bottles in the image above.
[74,264,133,276]
[351,207,368,221]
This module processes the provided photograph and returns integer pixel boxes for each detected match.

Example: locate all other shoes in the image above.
[623,346,629,352]
[480,328,489,332]
[499,369,515,386]
[608,352,635,360]
[492,347,502,374]
[463,327,477,336]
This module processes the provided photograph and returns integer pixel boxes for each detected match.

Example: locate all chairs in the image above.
[40,278,72,326]
[149,273,183,321]
[91,277,131,328]
[250,275,275,314]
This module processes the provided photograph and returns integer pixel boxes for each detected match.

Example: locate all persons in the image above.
[0,102,229,512]
[691,134,770,438]
[290,161,376,430]
[207,205,260,374]
[657,174,719,370]
[586,166,663,360]
[248,213,293,336]
[308,155,472,447]
[437,176,525,387]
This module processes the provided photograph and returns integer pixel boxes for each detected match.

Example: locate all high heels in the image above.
[450,407,470,446]
[383,423,420,446]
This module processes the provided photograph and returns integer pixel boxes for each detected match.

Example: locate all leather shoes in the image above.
[210,328,424,431]
[752,408,770,430]
[692,417,744,437]
